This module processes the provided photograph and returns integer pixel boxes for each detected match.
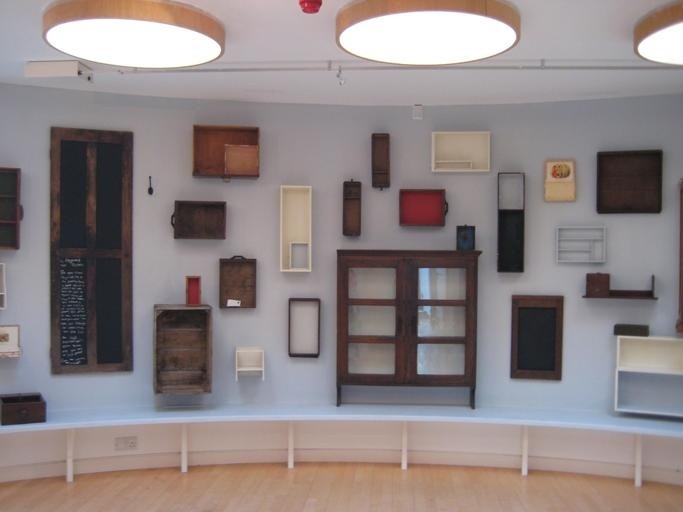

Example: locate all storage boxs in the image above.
[0,393,46,425]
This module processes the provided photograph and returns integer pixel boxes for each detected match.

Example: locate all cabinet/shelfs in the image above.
[171,200,230,242]
[185,275,202,306]
[218,255,259,310]
[0,263,7,310]
[287,297,320,359]
[429,131,491,176]
[371,133,392,190]
[335,248,483,409]
[456,224,475,249]
[192,125,261,182]
[399,188,450,229]
[153,302,213,395]
[556,226,607,264]
[342,180,363,237]
[234,345,265,384]
[0,167,21,249]
[278,184,314,273]
[596,148,662,214]
[613,334,683,419]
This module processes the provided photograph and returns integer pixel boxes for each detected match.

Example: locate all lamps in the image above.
[335,0,520,66]
[41,0,226,70]
[633,3,683,66]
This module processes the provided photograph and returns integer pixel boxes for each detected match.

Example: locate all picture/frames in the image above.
[0,325,20,353]
[510,294,564,380]
